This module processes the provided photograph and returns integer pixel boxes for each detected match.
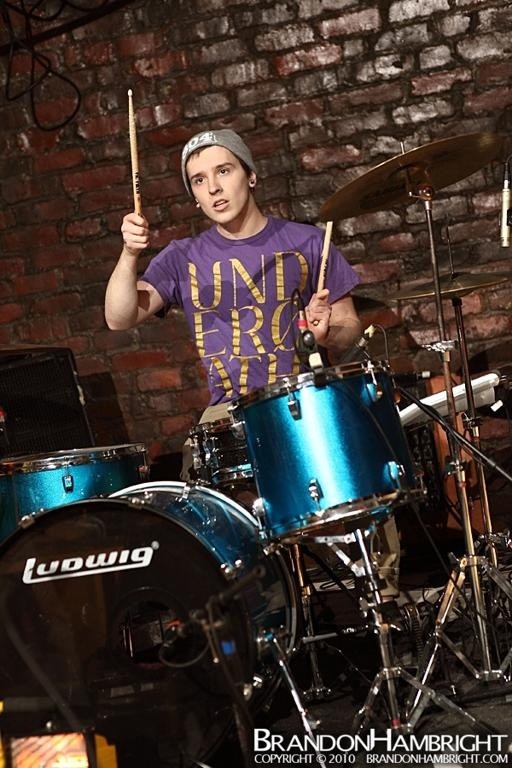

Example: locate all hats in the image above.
[180,128,257,198]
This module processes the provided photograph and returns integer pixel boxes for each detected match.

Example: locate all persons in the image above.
[104,129,363,481]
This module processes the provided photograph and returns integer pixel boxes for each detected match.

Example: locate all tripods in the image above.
[263,542,375,715]
[343,526,505,755]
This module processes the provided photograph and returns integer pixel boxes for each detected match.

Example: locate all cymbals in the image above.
[317,128,510,221]
[383,268,511,299]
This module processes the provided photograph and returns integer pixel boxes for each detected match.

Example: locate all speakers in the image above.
[1,347,98,458]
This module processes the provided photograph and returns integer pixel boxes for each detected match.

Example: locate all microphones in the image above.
[294,293,318,355]
[334,320,380,368]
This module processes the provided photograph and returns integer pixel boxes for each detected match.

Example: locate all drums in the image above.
[192,419,255,491]
[1,480,298,768]
[2,446,154,533]
[227,356,423,541]
